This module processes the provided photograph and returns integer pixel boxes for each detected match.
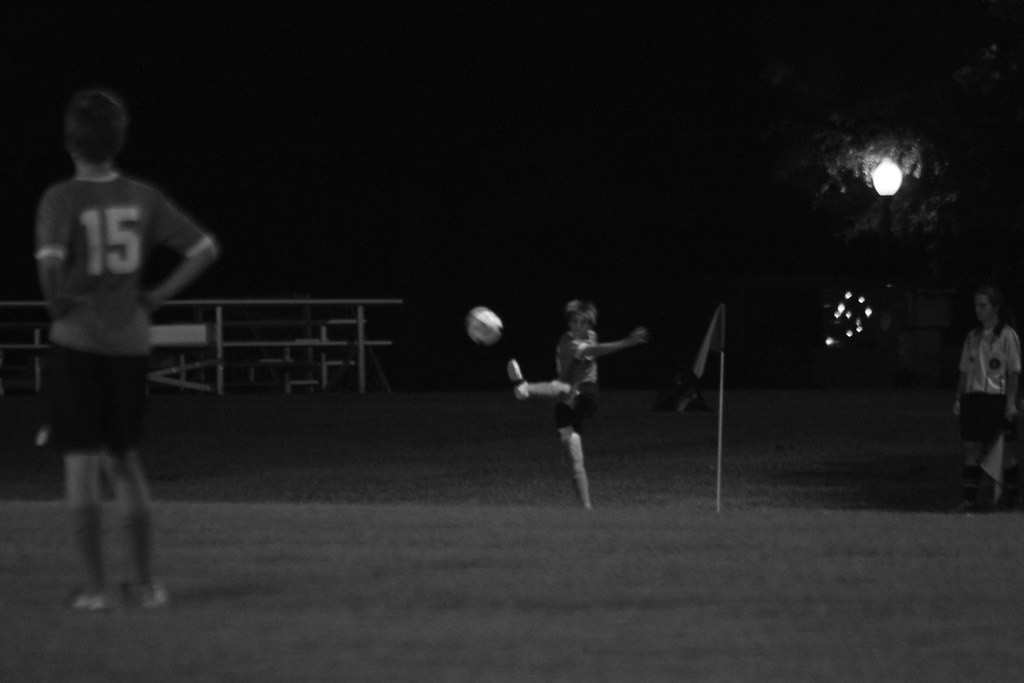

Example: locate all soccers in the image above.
[468,307,503,343]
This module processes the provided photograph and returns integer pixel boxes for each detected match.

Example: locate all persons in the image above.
[954,283,1023,507]
[33,88,219,618]
[507,300,646,509]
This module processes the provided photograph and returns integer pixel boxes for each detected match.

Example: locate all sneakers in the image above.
[107,578,159,609]
[507,358,529,400]
[43,581,107,611]
